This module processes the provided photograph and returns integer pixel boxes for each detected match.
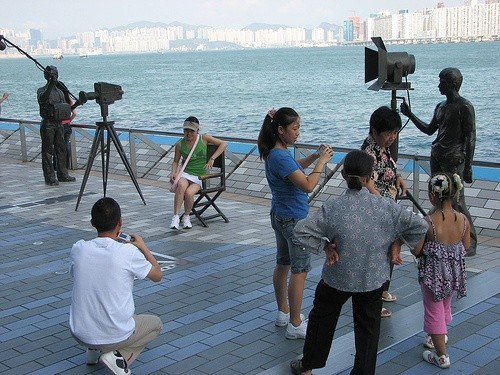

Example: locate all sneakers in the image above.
[423,333,448,349]
[284,319,307,340]
[99,350,133,375]
[86,347,105,365]
[169,215,180,230]
[181,214,192,229]
[423,350,451,368]
[275,309,305,326]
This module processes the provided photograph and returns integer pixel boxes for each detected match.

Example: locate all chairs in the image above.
[179,143,229,228]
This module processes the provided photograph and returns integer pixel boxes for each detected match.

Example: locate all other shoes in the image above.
[45,179,59,186]
[56,175,76,182]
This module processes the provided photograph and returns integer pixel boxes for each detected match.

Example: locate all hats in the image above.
[182,116,199,132]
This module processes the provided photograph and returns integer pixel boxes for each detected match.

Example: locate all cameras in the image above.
[117,232,132,241]
[319,146,330,154]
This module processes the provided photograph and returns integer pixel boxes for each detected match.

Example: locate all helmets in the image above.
[44,65,59,80]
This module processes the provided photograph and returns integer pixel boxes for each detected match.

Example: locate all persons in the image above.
[67,197,163,375]
[291,149,430,375]
[168,116,227,230]
[257,107,335,341]
[35,65,76,186]
[53,95,76,170]
[346,105,407,317]
[411,172,472,369]
[400,67,477,260]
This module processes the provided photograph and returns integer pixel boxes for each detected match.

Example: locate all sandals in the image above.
[290,360,313,375]
[382,290,397,302]
[380,307,392,317]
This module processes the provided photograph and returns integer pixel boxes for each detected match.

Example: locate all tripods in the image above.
[74,102,147,212]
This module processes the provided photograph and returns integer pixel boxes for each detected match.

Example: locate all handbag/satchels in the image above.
[169,164,182,193]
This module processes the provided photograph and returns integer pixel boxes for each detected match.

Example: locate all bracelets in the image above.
[210,157,215,160]
